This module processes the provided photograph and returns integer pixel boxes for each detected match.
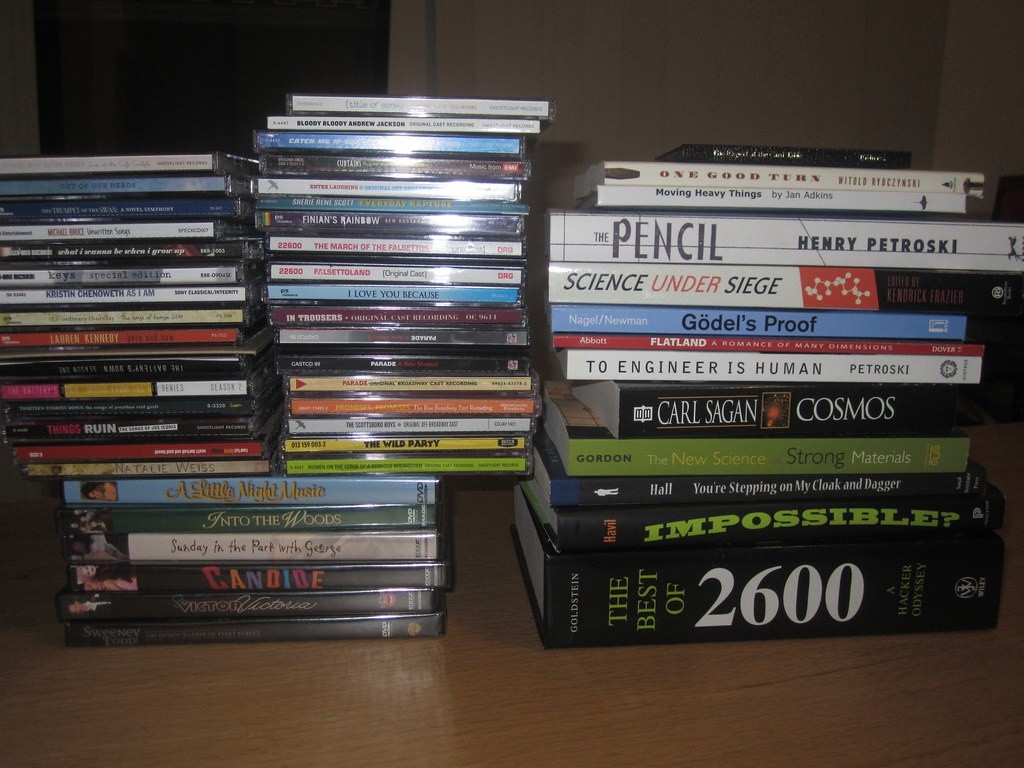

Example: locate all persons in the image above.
[65,481,138,618]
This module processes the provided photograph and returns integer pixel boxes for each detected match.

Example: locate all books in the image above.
[505,141,1024,651]
